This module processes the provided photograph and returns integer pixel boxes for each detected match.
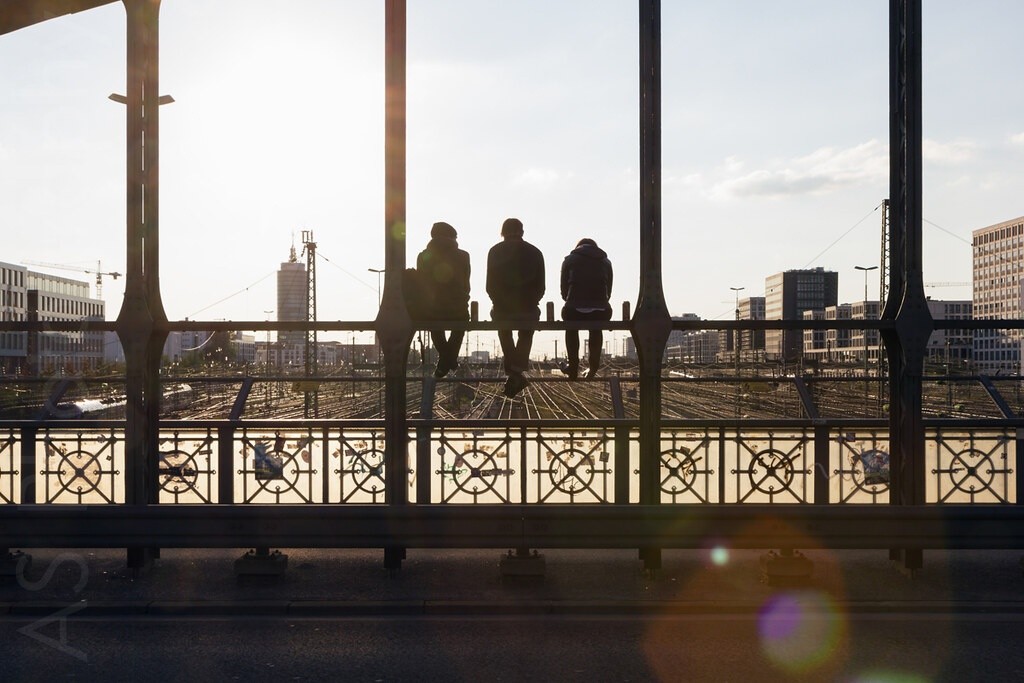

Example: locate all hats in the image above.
[430,221,460,242]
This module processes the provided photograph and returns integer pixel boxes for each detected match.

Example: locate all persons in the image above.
[417,221,470,379]
[486,218,546,398]
[560,239,613,379]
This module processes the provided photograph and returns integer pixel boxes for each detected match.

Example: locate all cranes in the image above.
[19,259,123,300]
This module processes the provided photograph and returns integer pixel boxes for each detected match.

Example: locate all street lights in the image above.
[731,287,746,310]
[854,266,878,372]
[369,268,386,308]
[264,310,274,375]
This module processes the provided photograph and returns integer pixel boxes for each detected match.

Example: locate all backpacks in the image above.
[403,267,436,322]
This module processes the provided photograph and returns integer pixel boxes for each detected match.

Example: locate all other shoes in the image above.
[504,373,530,399]
[582,368,595,382]
[559,361,578,381]
